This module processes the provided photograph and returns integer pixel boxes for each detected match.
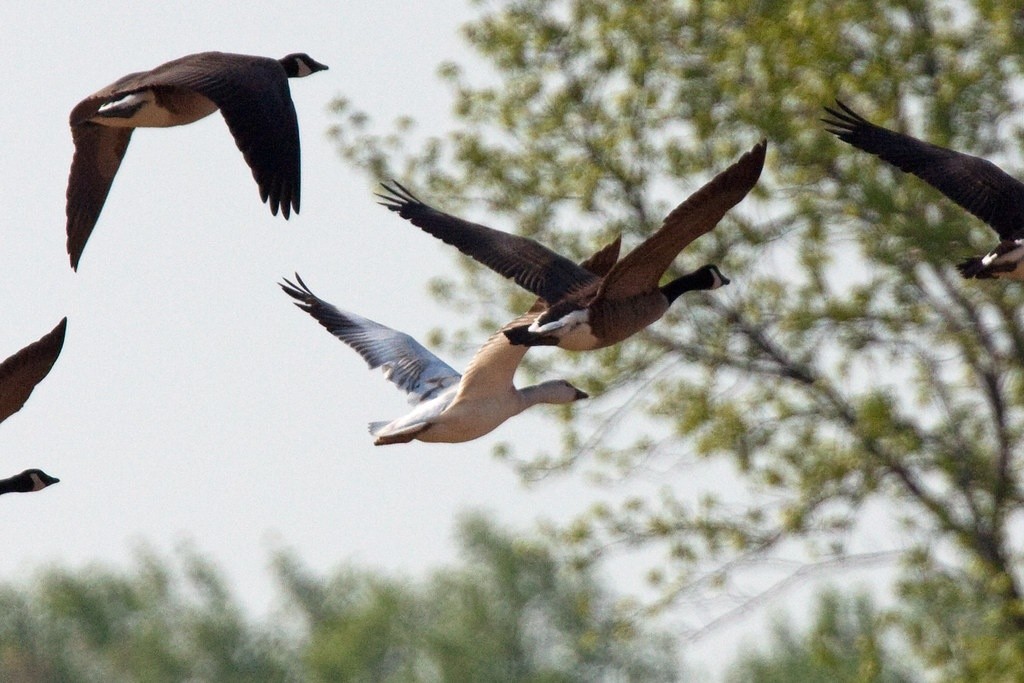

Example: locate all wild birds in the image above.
[816,96,1024,282]
[0,315,67,495]
[275,136,770,447]
[64,50,330,273]
